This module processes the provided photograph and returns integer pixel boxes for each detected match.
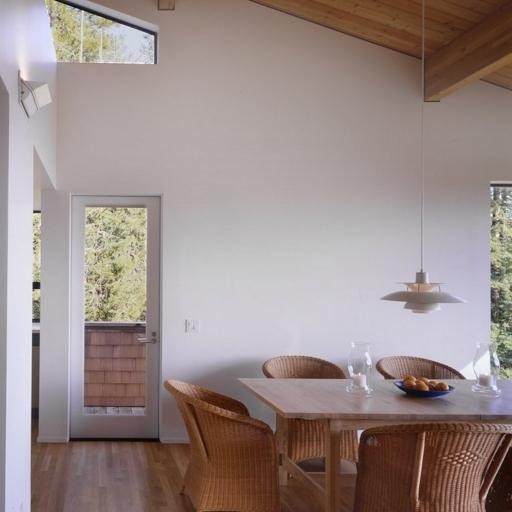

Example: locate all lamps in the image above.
[380,2,467,313]
[18,69,52,120]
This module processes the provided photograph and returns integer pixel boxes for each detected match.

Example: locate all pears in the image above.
[403,375,448,390]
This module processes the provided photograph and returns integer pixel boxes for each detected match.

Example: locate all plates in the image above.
[393,378,456,398]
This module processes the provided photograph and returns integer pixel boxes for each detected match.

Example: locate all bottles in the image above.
[346,340,373,397]
[471,343,502,397]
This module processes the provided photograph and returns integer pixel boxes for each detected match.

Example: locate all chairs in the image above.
[281,418,511,512]
[162,375,285,512]
[374,355,469,380]
[258,355,361,473]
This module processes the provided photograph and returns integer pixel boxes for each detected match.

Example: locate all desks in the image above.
[236,376,510,510]
